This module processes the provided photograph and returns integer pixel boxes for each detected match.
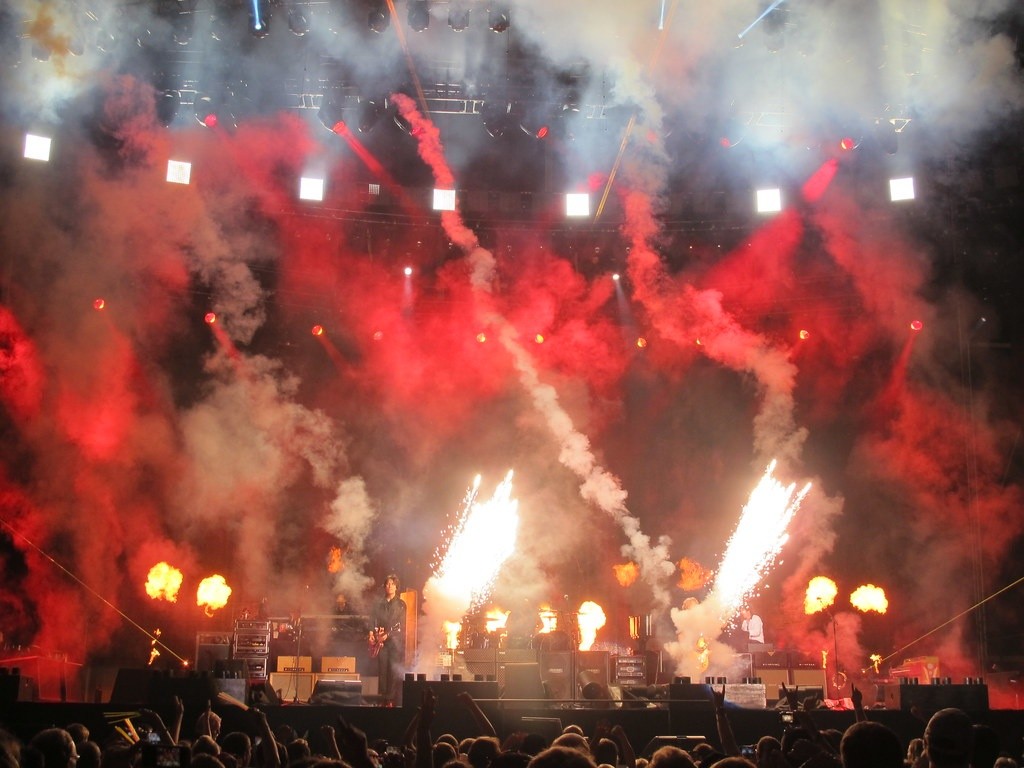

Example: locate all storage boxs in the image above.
[316,673,360,684]
[270,673,314,704]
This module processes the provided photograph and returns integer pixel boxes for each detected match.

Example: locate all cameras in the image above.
[742,745,755,756]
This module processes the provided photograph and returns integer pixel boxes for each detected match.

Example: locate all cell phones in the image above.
[141,744,191,768]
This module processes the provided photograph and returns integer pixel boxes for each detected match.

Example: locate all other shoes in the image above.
[378,702,384,707]
[387,702,392,707]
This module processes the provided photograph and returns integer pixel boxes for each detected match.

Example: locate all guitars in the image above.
[366,622,401,657]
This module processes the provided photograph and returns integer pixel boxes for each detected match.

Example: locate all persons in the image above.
[365,575,408,707]
[330,594,367,658]
[0,684,1024,768]
[676,597,709,684]
[740,606,764,645]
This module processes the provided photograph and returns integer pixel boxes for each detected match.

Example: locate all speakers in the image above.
[269,671,362,705]
[521,717,563,740]
[0,675,37,702]
[665,683,715,710]
[748,643,827,708]
[431,648,609,708]
[640,736,706,761]
[110,630,248,710]
